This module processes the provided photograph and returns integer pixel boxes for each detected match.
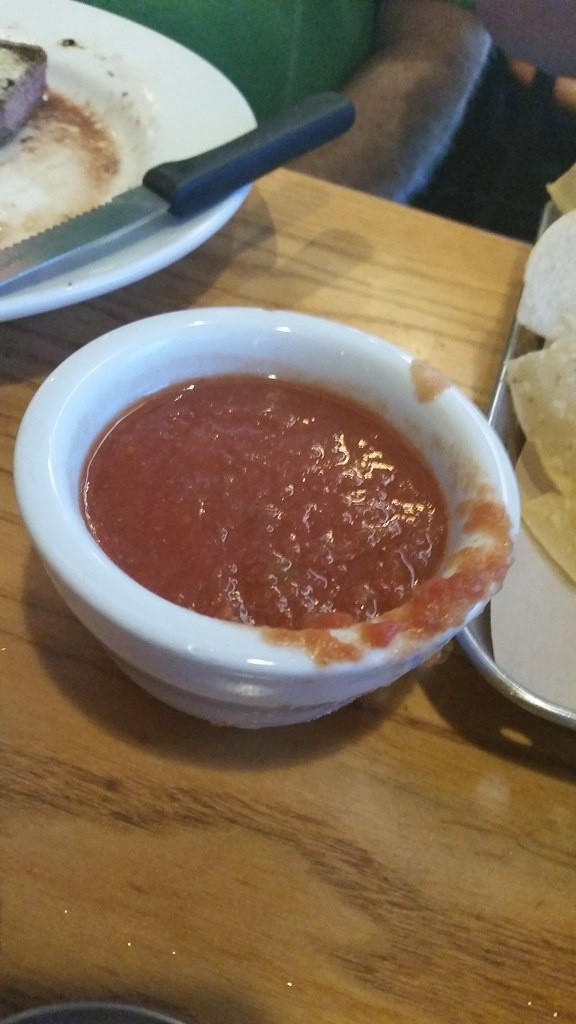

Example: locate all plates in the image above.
[455,201,576,726]
[0,0,257,323]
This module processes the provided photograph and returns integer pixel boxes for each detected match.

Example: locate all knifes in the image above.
[0,94,353,289]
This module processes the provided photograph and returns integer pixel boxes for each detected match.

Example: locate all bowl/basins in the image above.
[13,305,521,726]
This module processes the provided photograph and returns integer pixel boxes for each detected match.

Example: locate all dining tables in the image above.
[0,162,576,1024]
